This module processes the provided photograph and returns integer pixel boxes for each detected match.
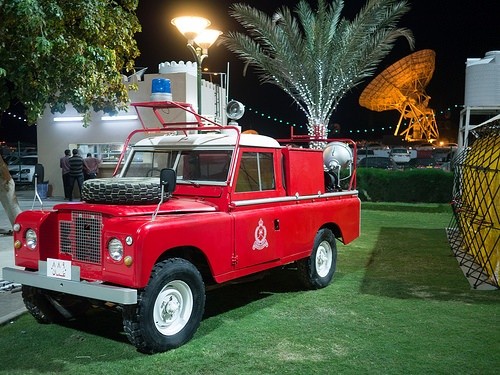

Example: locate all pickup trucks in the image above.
[3,101,362,354]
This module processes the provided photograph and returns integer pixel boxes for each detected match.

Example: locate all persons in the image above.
[60,149,98,202]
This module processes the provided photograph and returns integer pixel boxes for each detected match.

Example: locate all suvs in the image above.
[8,155,38,188]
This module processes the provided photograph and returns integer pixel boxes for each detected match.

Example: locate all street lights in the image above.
[170,15,224,131]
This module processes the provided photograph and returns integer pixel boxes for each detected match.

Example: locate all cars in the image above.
[353,142,460,170]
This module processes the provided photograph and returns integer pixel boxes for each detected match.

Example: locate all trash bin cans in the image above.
[37,181,48,199]
